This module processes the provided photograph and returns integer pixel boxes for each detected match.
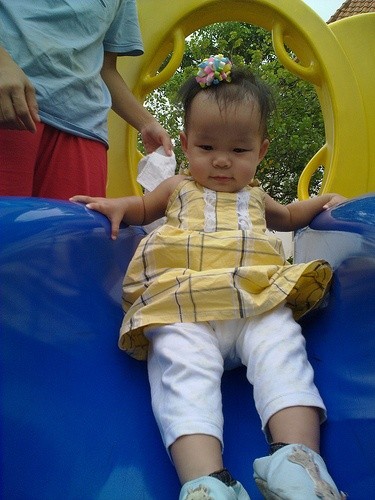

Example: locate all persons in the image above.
[0,0,174,201]
[69,56,351,500]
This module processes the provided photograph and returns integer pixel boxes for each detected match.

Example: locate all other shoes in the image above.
[253,441,347,500]
[178,468,252,500]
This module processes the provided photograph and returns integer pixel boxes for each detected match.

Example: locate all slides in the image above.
[0,193,375,500]
